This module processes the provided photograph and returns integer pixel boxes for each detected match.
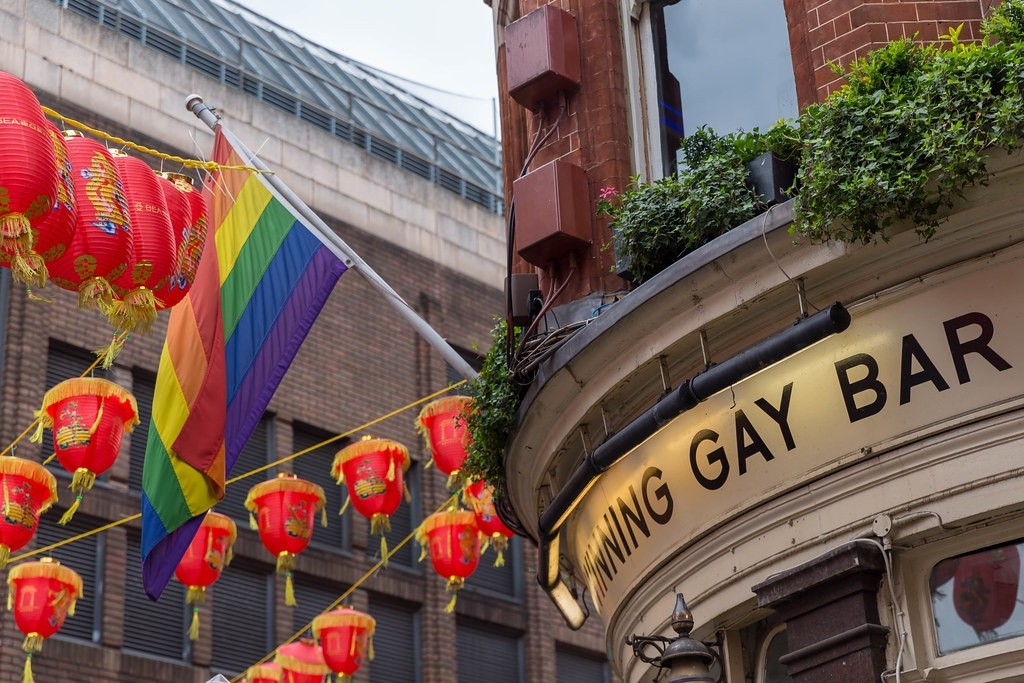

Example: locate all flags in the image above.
[138,122,356,606]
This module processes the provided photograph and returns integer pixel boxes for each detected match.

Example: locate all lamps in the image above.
[625,593,728,683]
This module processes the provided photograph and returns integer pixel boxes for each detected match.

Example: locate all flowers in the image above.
[596,124,781,285]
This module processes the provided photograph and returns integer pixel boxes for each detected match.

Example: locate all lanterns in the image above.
[951,544,1021,643]
[0,70,210,330]
[0,375,518,683]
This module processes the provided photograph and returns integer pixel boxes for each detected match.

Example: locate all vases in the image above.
[611,150,790,283]
